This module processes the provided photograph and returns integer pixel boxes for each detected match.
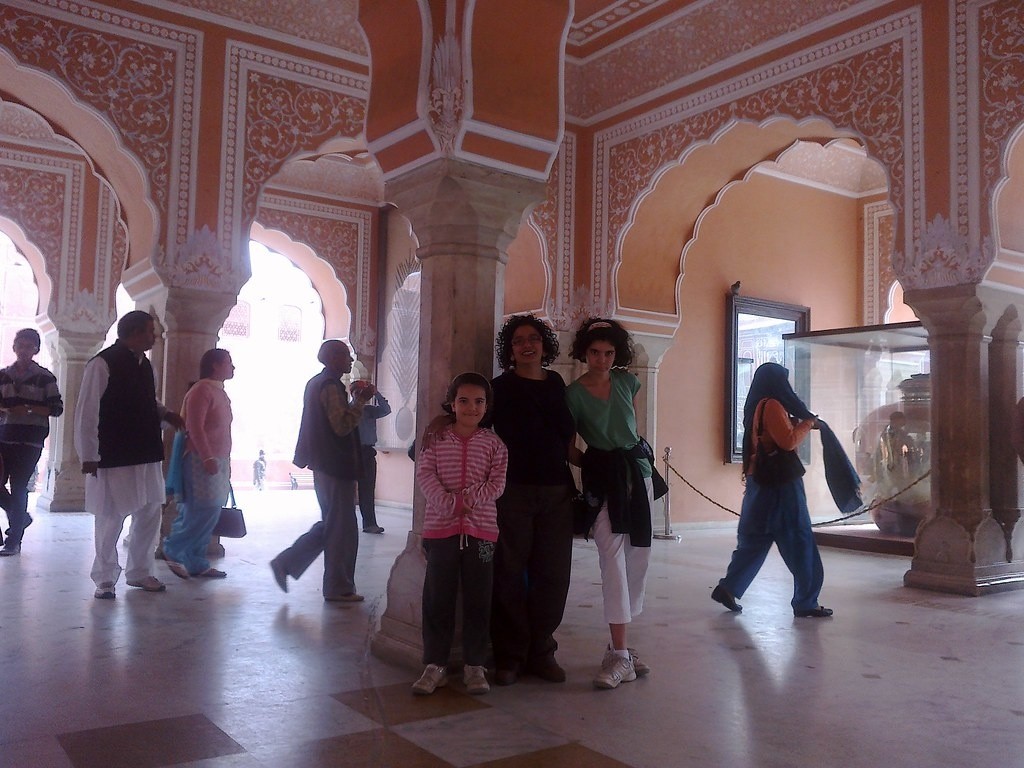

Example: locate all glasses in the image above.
[510,333,542,346]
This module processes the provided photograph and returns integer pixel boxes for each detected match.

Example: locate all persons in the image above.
[420,314,576,686]
[73,311,189,600]
[411,373,508,697]
[564,317,655,690]
[874,412,918,505]
[348,381,393,533]
[711,363,833,617]
[0,328,64,556]
[161,349,235,581]
[271,339,374,601]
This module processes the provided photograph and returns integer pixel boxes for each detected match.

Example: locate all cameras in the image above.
[359,387,374,401]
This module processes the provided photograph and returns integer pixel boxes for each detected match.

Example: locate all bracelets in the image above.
[25,404,33,416]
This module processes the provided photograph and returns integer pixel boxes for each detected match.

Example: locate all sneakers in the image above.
[592,650,637,688]
[602,643,650,675]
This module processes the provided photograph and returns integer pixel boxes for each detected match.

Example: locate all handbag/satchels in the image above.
[212,481,247,538]
[753,399,807,487]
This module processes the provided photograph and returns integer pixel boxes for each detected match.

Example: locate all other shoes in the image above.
[95,581,116,600]
[324,592,364,601]
[5,513,32,535]
[157,546,187,578]
[793,606,834,618]
[710,585,743,611]
[462,664,490,694]
[272,560,288,591]
[187,568,226,577]
[533,651,566,682]
[411,663,449,694]
[363,524,384,532]
[495,669,518,685]
[0,542,21,556]
[127,568,166,592]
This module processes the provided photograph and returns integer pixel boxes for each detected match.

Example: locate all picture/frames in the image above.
[725,280,810,465]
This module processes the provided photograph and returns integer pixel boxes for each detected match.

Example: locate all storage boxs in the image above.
[782,320,931,557]
